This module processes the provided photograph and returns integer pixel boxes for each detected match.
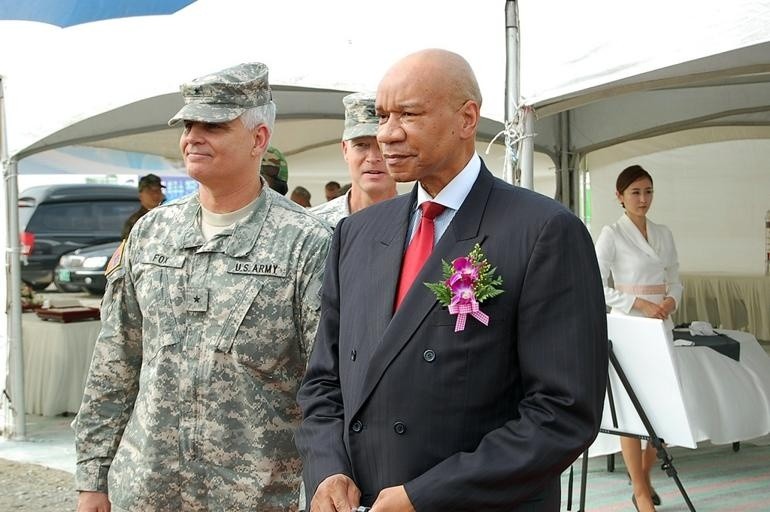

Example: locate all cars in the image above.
[54,239,122,297]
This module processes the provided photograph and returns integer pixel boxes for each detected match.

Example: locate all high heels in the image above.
[628,473,660,512]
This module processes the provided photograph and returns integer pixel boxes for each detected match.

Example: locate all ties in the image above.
[394,202,445,317]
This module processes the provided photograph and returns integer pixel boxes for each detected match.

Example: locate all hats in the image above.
[168,62,272,126]
[138,175,166,192]
[342,92,380,141]
[260,145,287,182]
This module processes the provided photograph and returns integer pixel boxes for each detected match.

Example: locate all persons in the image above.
[325,180,341,202]
[70,61,333,511]
[259,146,289,196]
[293,47,611,512]
[303,92,399,224]
[593,163,684,512]
[290,186,312,209]
[120,173,167,242]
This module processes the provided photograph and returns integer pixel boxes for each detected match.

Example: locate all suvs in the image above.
[19,184,149,291]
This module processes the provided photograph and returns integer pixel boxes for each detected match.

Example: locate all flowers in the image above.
[423,243,505,333]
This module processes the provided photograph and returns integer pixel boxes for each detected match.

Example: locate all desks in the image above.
[672,324,755,452]
[20,311,103,418]
